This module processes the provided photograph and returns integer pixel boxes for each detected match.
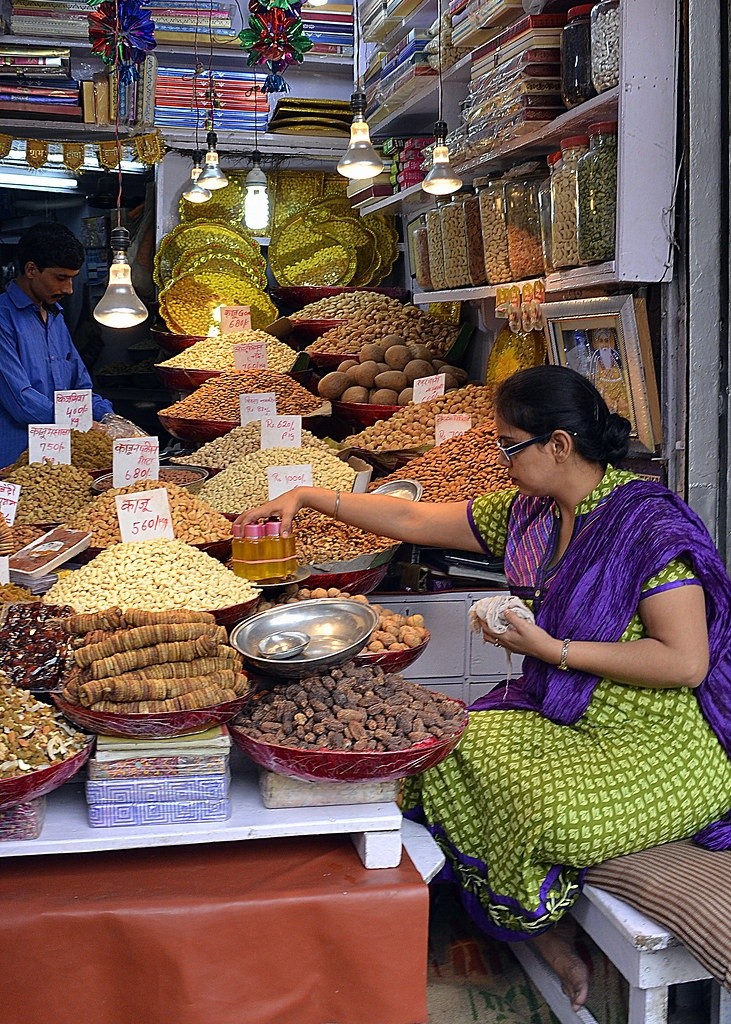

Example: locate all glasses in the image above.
[497,430,577,462]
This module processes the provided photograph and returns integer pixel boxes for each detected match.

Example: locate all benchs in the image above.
[510,886,730,1024]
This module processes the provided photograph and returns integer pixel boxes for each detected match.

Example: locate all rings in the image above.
[493,637,500,648]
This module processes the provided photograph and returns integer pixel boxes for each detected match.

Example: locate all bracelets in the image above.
[332,490,340,521]
[556,639,573,672]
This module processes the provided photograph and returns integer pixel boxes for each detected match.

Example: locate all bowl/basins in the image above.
[152,365,224,386]
[306,350,361,370]
[156,414,327,443]
[91,466,209,497]
[227,698,468,782]
[229,598,380,676]
[150,325,208,353]
[0,738,98,810]
[334,400,409,426]
[49,679,259,738]
[273,284,407,305]
[291,319,351,339]
[258,631,310,657]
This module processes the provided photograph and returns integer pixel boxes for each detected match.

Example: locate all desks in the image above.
[1,839,428,1024]
[97,387,175,402]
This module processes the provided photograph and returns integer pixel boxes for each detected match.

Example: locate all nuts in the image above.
[411,131,618,291]
[0,292,529,780]
[560,3,619,108]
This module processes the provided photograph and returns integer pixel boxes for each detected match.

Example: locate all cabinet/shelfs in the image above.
[0,34,354,150]
[355,0,682,304]
[370,593,525,707]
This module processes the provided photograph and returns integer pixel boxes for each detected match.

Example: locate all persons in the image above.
[1,222,118,470]
[232,363,731,1014]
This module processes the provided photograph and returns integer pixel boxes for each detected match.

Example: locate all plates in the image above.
[367,477,425,504]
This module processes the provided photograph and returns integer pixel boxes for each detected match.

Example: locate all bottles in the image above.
[243,525,266,581]
[590,0,619,95]
[232,524,246,579]
[479,171,513,285]
[551,136,589,269]
[502,150,563,281]
[576,121,619,264]
[559,4,595,109]
[413,176,487,291]
[278,522,298,574]
[263,522,286,577]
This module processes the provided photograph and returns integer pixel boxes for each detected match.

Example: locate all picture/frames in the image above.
[540,294,657,458]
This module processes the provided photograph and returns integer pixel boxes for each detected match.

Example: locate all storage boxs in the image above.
[464,13,565,157]
[364,27,437,123]
[382,135,434,195]
